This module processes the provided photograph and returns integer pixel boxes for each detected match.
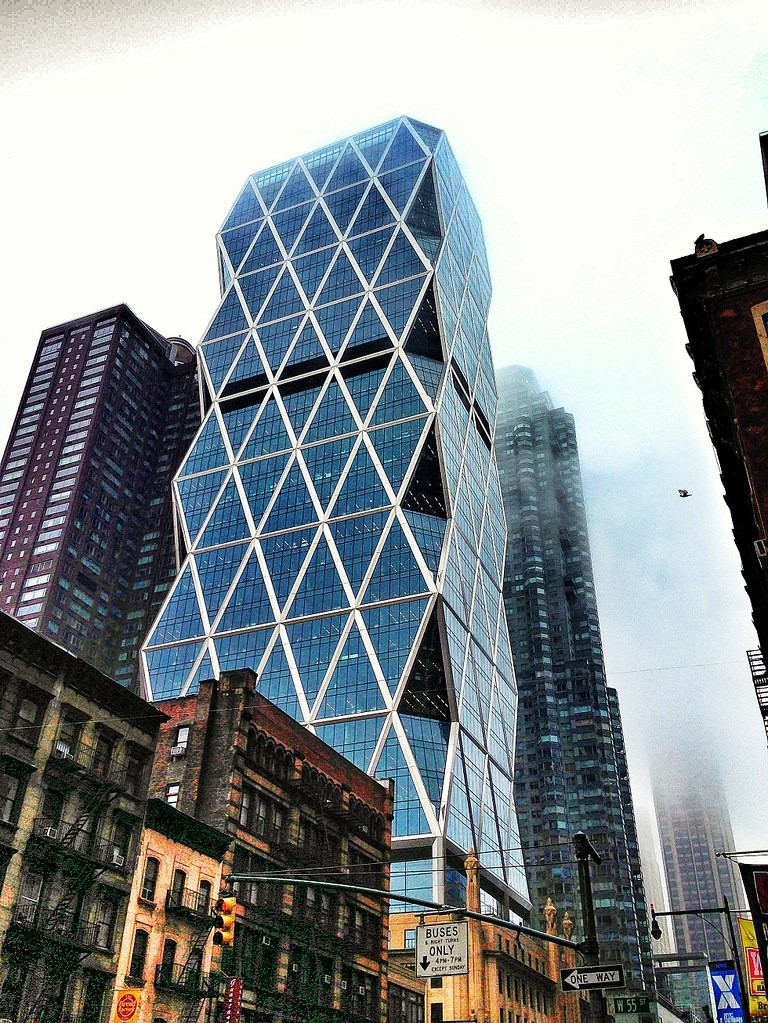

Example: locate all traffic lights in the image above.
[213,897,236,947]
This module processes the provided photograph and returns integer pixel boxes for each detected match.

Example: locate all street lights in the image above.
[650,894,752,1023]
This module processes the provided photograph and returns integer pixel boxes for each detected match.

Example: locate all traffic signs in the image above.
[415,920,469,978]
[560,964,627,992]
[606,996,649,1015]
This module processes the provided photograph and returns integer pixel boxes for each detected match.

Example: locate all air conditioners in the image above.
[321,974,331,984]
[261,936,271,947]
[292,964,299,973]
[356,986,365,996]
[170,746,185,756]
[43,827,57,839]
[340,981,347,990]
[107,855,124,867]
